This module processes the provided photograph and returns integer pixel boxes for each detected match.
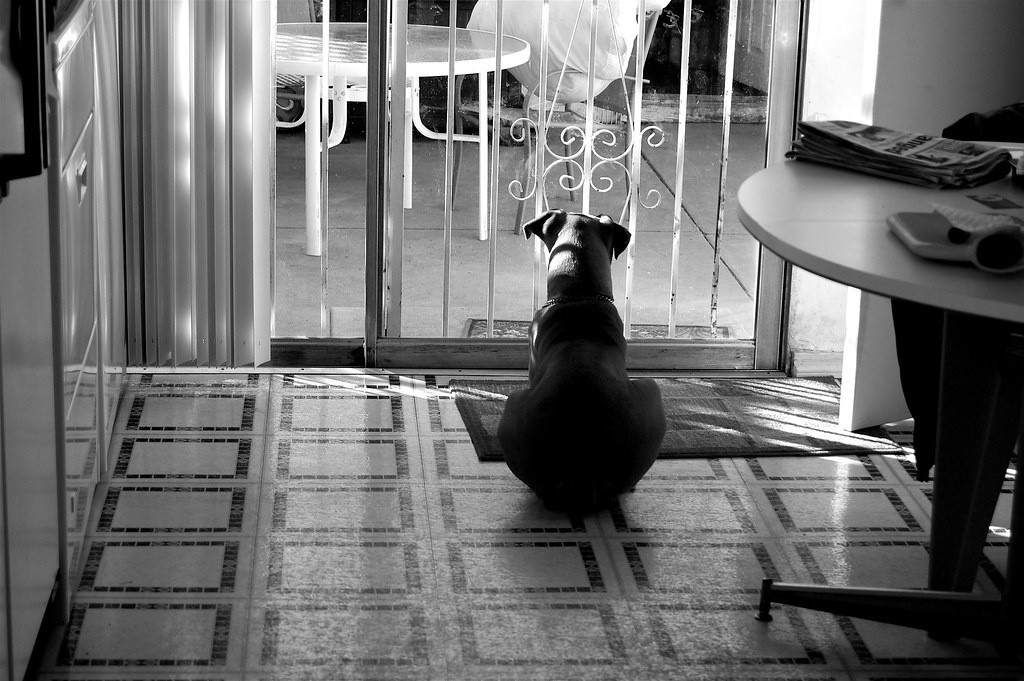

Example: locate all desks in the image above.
[276,21,530,257]
[738,139,1024,635]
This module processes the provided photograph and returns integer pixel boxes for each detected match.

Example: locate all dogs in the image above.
[496,208,667,516]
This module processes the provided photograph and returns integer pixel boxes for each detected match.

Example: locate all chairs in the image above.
[276,0,671,233]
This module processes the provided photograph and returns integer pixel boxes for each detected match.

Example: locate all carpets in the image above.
[438,376,900,463]
[463,317,729,338]
